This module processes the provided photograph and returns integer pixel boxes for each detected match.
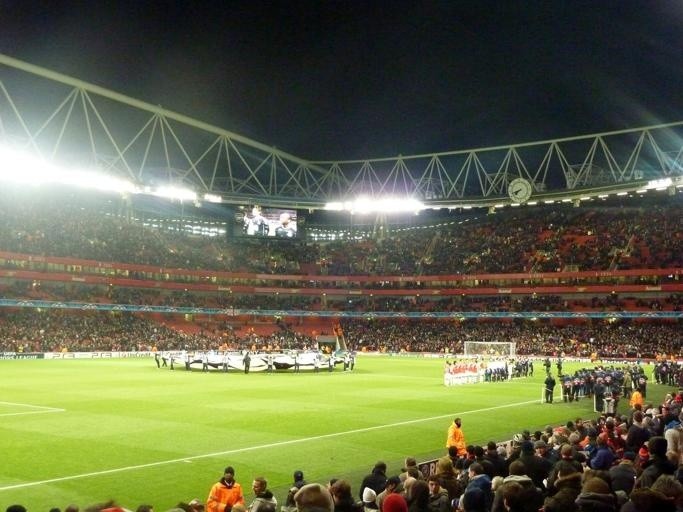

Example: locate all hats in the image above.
[294,472,302,481]
[383,493,408,512]
[649,437,667,452]
[362,486,377,502]
[534,441,549,450]
[294,483,334,512]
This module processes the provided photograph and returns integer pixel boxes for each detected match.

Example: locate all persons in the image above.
[0,189,682,375]
[201,375,682,511]
[5,499,201,511]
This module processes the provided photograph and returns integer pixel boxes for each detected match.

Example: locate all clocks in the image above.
[507,178,531,204]
